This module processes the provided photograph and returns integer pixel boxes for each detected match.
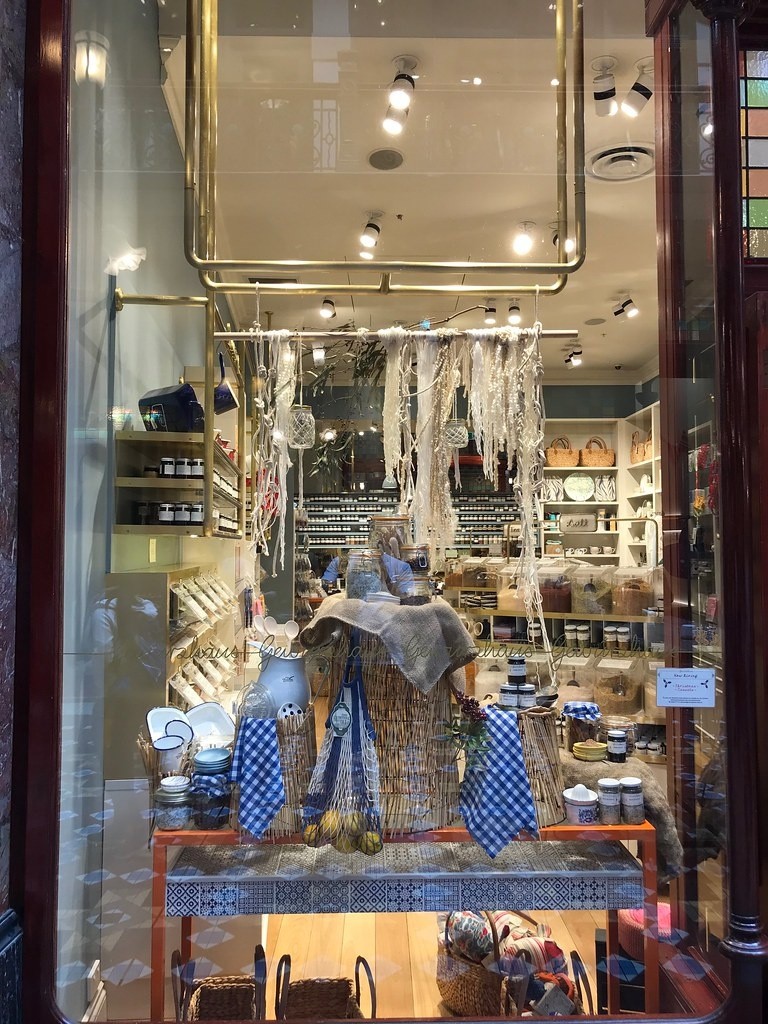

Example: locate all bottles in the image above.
[528,623,542,649]
[619,777,646,825]
[597,778,622,825]
[557,700,675,763]
[497,656,537,709]
[603,626,630,651]
[135,457,240,534]
[290,491,540,605]
[565,624,590,648]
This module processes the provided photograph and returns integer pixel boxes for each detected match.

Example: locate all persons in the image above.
[322,544,416,603]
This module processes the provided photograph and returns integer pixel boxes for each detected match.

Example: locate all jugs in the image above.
[247,640,331,713]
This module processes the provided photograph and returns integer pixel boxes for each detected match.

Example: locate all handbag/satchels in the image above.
[274,952,378,1022]
[170,943,265,1023]
[498,950,595,1020]
[437,904,539,1019]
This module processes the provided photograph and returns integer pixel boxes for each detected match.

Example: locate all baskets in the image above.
[581,435,615,467]
[545,436,579,467]
[310,672,329,697]
[628,427,653,464]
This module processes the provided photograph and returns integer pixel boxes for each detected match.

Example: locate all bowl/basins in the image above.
[186,701,236,749]
[160,776,191,792]
[146,705,192,744]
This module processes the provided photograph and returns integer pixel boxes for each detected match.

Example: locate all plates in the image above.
[640,473,651,486]
[542,472,616,504]
[193,747,232,774]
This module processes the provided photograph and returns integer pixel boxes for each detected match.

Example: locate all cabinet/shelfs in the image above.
[297,490,525,549]
[622,400,663,595]
[104,289,261,781]
[453,608,665,702]
[540,417,622,570]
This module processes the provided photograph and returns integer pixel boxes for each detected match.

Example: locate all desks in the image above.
[151,815,661,1024]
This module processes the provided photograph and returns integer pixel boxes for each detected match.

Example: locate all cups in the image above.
[165,720,193,746]
[463,618,491,639]
[563,546,616,555]
[153,734,185,773]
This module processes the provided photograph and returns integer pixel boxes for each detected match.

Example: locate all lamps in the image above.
[359,210,383,260]
[590,55,621,117]
[446,342,468,446]
[508,298,523,324]
[383,54,420,134]
[286,341,314,448]
[620,289,638,317]
[611,297,627,322]
[311,342,325,365]
[483,297,497,324]
[561,339,582,369]
[382,471,398,490]
[319,295,336,319]
[621,56,655,116]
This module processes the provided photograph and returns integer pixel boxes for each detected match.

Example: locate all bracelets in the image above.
[386,575,392,584]
